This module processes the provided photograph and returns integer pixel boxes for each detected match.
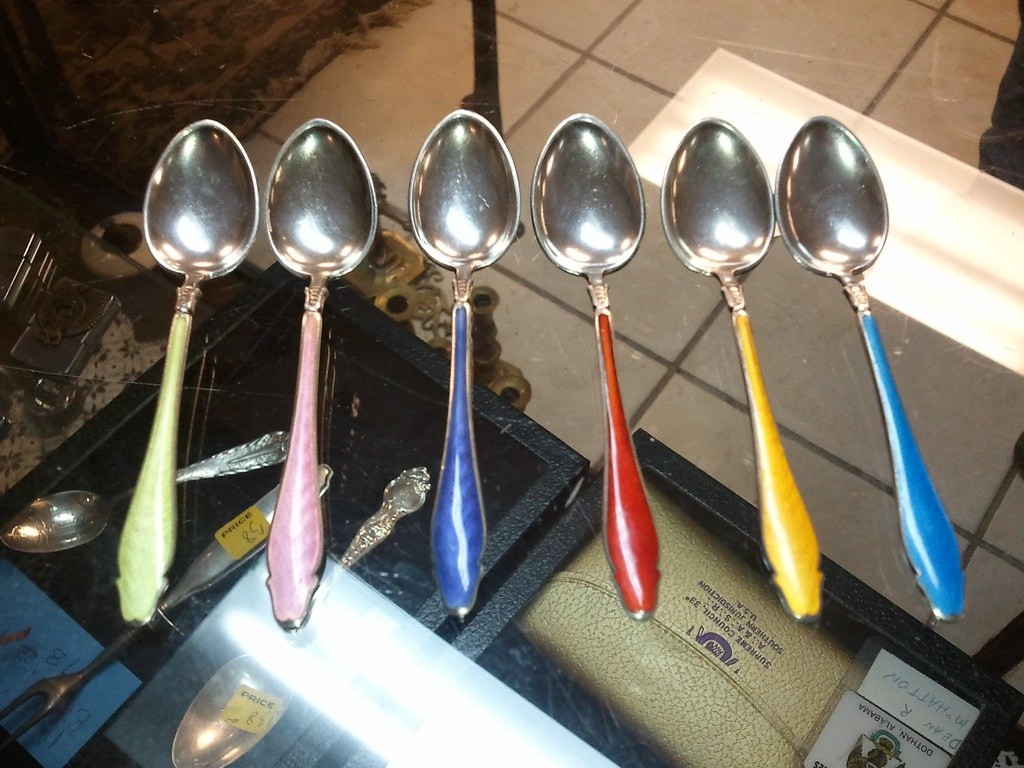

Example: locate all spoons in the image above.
[660,119,824,620]
[0,431,291,552]
[115,120,259,628]
[530,113,659,620]
[172,467,433,768]
[773,115,966,623]
[263,117,378,631]
[407,110,519,620]
